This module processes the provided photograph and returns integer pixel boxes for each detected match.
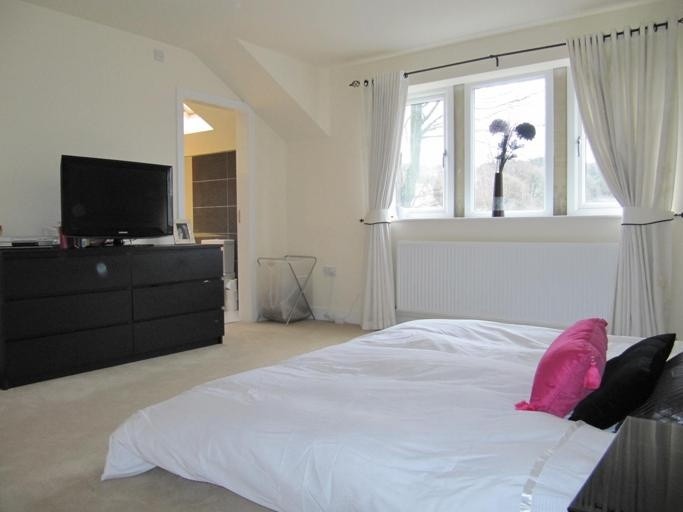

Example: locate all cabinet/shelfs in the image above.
[1,244,224,391]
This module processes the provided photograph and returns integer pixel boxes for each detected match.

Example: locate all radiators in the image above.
[395,239,620,335]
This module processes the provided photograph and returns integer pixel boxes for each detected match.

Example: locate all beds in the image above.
[98,316,682,512]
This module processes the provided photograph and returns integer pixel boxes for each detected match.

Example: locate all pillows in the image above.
[513,318,608,418]
[568,332,683,429]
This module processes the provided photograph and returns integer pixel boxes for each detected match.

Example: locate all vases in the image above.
[493,173,505,217]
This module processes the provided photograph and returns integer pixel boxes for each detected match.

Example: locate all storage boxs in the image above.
[200,239,236,279]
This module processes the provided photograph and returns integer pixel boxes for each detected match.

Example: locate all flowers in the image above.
[489,119,535,172]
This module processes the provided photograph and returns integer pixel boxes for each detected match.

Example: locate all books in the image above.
[11,240,58,246]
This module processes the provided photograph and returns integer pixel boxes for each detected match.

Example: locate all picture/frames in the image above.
[173,219,196,244]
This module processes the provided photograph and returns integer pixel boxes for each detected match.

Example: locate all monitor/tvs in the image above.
[60,155,173,249]
